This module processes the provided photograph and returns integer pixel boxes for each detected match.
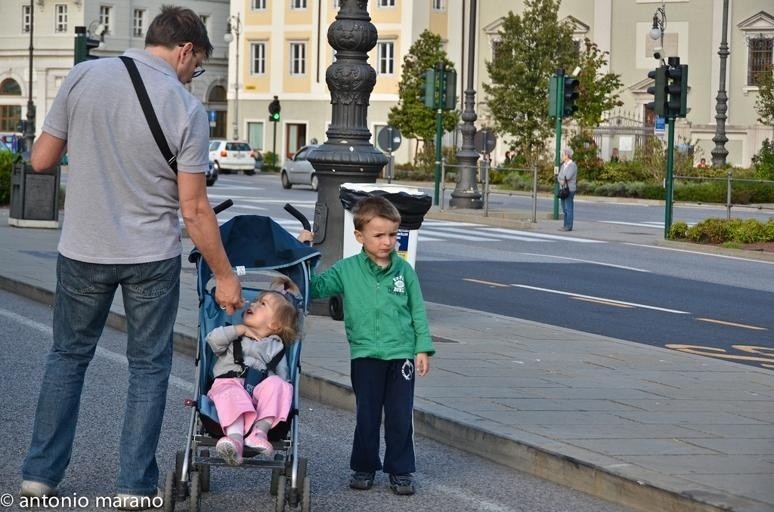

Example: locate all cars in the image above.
[203,160,220,186]
[280,144,320,191]
[208,140,256,175]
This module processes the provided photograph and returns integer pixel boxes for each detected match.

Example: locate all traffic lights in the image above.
[74,36,101,66]
[272,100,280,121]
[664,68,681,114]
[563,77,579,119]
[645,71,656,111]
[419,71,433,107]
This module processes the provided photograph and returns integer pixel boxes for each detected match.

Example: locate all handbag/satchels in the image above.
[558,188,569,198]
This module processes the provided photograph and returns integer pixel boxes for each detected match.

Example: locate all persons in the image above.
[696,157,710,168]
[554,143,580,232]
[296,194,438,495]
[18,6,248,511]
[202,290,300,468]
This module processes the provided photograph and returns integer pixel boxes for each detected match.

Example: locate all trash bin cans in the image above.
[328,182,432,320]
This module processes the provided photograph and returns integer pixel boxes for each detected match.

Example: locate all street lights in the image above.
[649,2,667,68]
[224,13,241,142]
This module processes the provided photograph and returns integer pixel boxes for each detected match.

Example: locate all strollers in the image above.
[168,200,322,512]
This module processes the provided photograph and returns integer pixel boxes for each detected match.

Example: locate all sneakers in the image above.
[244,424,274,456]
[18,479,60,501]
[215,436,244,467]
[389,472,418,495]
[349,471,375,490]
[116,486,164,511]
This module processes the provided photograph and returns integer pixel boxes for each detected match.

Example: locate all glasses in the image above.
[179,42,205,77]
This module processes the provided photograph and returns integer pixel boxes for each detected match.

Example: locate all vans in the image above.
[0,133,21,155]
